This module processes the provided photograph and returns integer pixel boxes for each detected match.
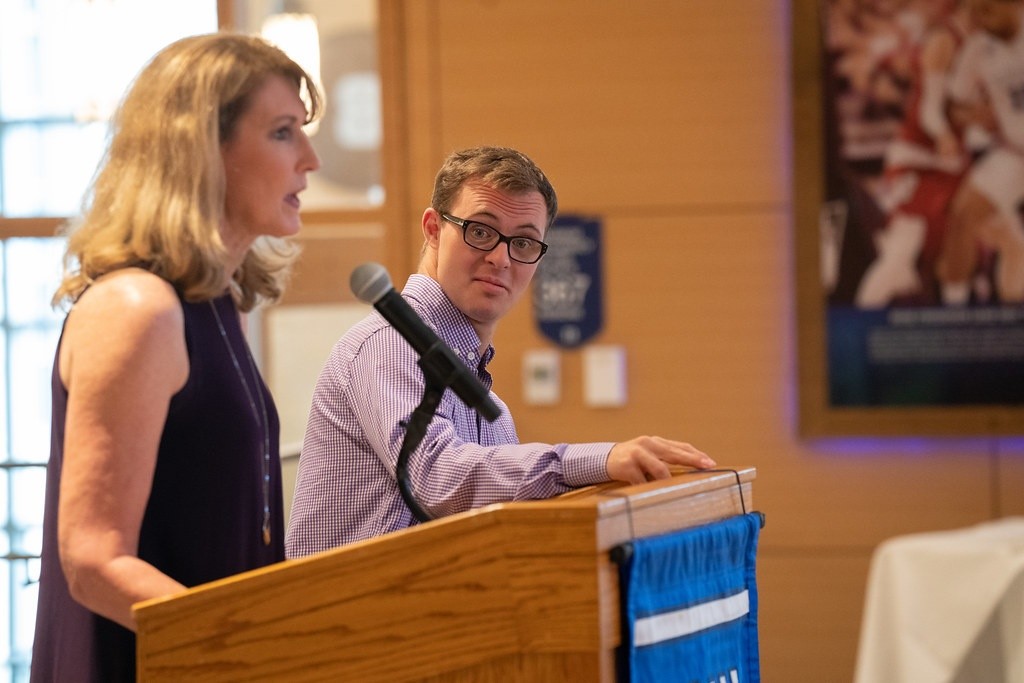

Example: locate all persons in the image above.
[282,147,720,560]
[28,33,326,683]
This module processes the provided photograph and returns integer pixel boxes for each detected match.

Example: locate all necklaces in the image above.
[204,290,271,545]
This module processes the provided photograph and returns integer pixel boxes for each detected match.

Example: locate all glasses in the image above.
[442,213,549,264]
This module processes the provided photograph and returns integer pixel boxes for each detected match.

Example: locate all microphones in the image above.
[350,262,502,424]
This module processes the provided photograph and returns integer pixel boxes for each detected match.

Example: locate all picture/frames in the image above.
[788,0,1024,442]
